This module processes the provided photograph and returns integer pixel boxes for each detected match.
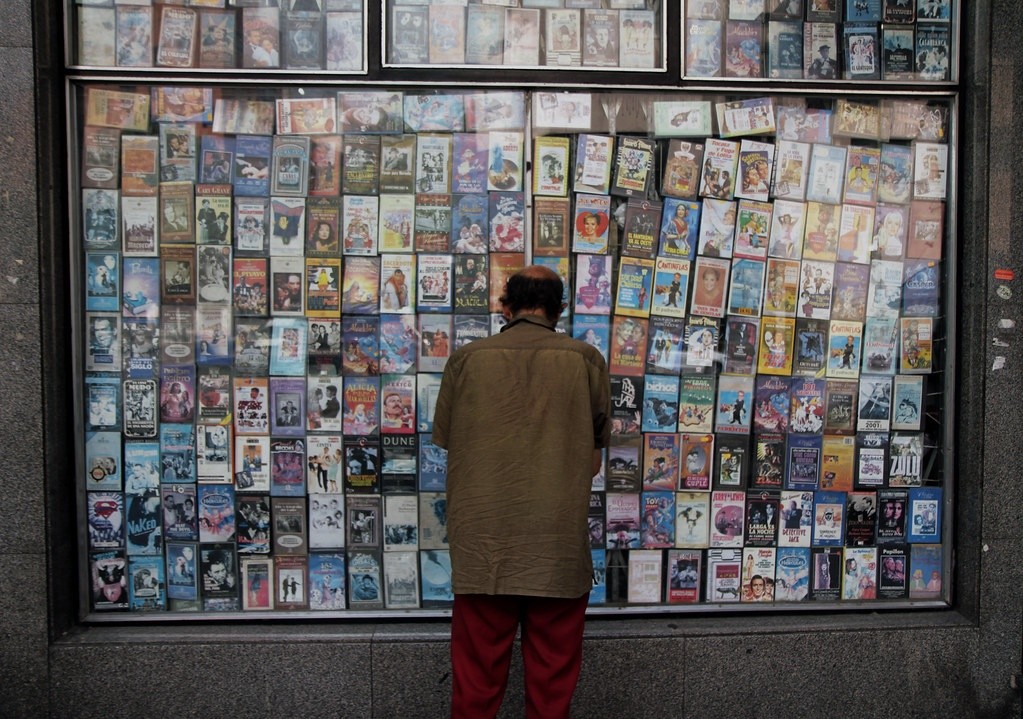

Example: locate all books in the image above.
[57,0,952,611]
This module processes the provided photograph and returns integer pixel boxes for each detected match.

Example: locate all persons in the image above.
[94,318,118,356]
[236,503,253,540]
[778,213,801,257]
[431,266,612,719]
[261,33,279,67]
[309,324,319,350]
[874,211,904,260]
[918,49,934,78]
[862,496,876,545]
[162,204,185,233]
[164,495,176,527]
[328,322,340,348]
[846,500,875,545]
[308,385,323,430]
[931,44,948,81]
[244,29,269,67]
[321,385,340,417]
[808,45,836,79]
[310,223,337,250]
[124,464,154,495]
[181,499,195,528]
[248,501,270,540]
[318,326,330,350]
